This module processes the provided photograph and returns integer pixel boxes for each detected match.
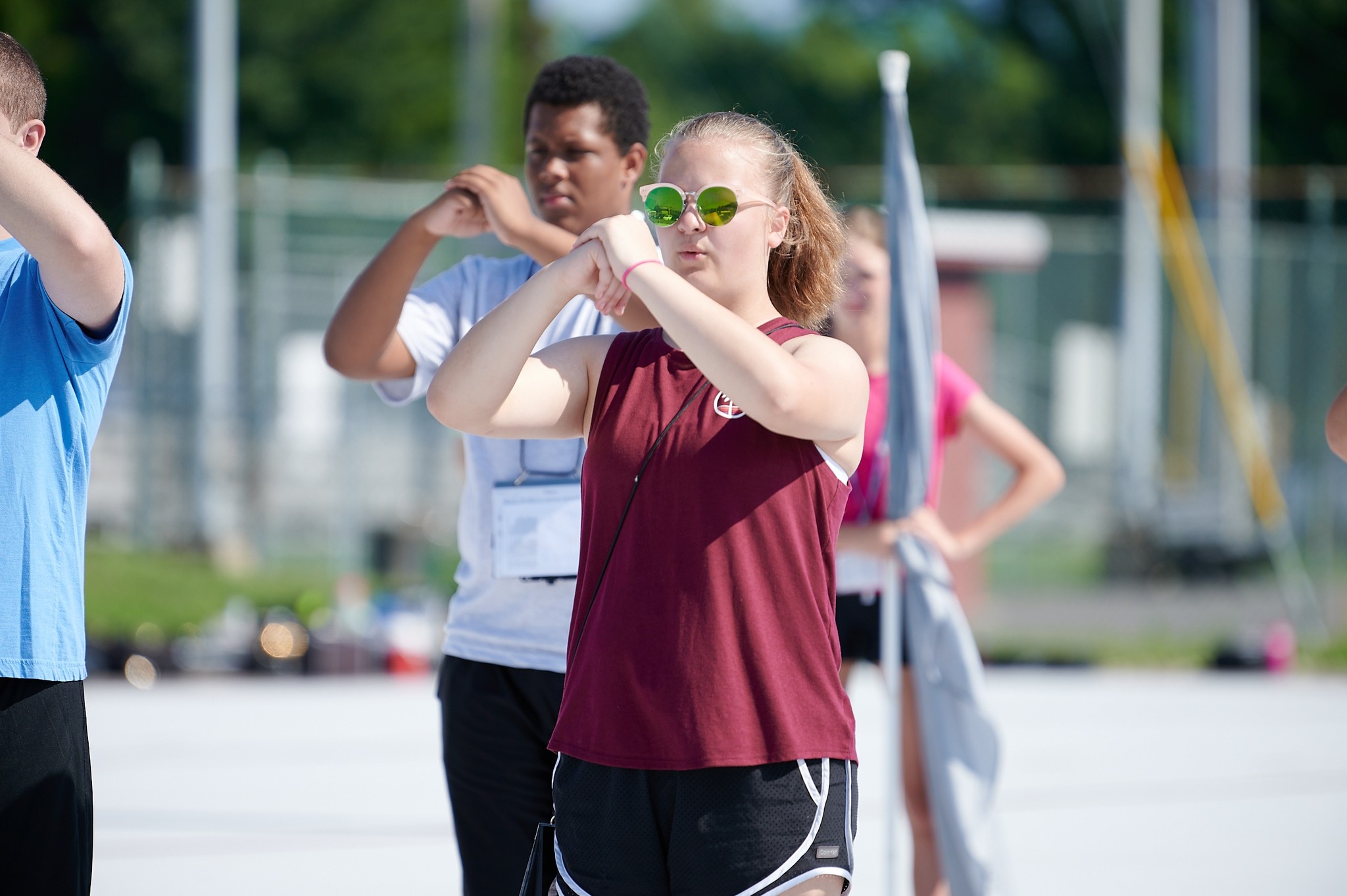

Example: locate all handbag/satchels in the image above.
[518,822,558,896]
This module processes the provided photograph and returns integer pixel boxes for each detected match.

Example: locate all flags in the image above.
[880,50,1008,896]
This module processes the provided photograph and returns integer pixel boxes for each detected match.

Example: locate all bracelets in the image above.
[623,260,663,292]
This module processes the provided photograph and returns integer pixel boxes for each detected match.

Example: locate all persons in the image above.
[0,31,135,896]
[811,208,1065,896]
[423,107,871,896]
[321,57,649,896]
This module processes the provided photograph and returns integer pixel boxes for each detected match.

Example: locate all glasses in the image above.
[639,182,779,228]
